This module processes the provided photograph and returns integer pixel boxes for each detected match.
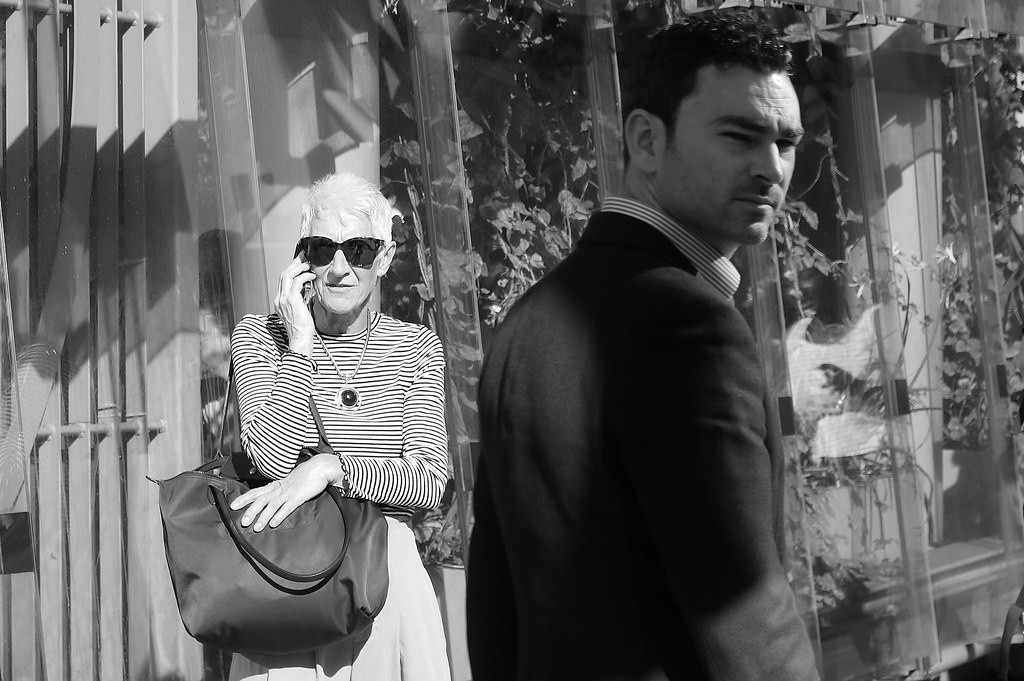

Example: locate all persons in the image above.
[463,10,818,680]
[777,216,895,477]
[223,169,453,680]
[201,225,279,460]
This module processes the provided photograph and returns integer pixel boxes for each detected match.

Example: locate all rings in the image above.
[275,479,283,487]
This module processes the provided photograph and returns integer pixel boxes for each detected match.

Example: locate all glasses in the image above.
[299,237,386,267]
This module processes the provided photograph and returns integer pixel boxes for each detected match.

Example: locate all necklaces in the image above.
[312,303,372,414]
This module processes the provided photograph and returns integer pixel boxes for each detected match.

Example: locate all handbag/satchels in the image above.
[146,321,389,655]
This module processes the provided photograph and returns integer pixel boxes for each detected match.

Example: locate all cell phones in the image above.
[300,269,312,304]
